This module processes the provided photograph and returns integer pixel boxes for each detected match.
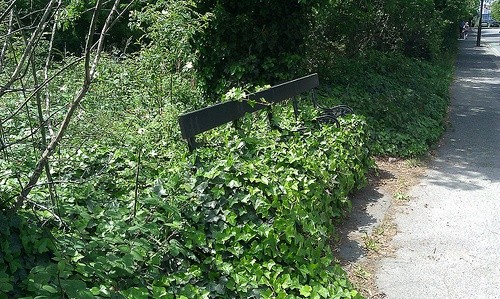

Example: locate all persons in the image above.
[462,21,469,39]
